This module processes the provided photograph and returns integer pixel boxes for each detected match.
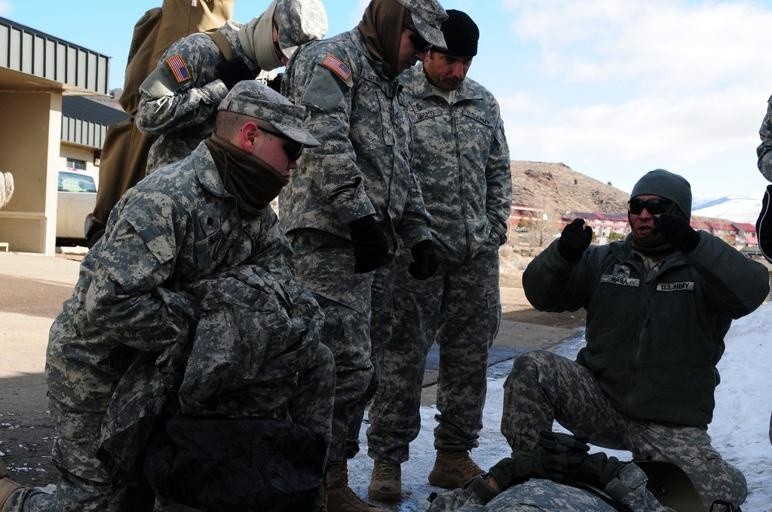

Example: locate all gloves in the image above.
[489,431,618,496]
[407,239,439,281]
[348,216,389,275]
[650,216,700,254]
[558,218,592,270]
[214,55,254,92]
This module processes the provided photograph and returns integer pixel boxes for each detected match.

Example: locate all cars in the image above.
[56,167,98,248]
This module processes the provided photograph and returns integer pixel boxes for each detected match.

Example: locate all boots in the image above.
[0,471,22,510]
[428,448,488,488]
[366,455,403,502]
[326,463,385,511]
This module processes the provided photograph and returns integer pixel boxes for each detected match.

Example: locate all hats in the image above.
[435,9,480,57]
[629,169,692,221]
[395,0,448,49]
[216,79,322,149]
[276,0,329,63]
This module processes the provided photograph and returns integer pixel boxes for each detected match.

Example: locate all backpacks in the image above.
[84,0,234,251]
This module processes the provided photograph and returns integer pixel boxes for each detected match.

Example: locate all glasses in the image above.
[626,197,674,216]
[241,121,304,161]
[400,22,431,50]
[271,22,288,60]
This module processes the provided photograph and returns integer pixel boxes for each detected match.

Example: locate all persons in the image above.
[755,94,772,267]
[424,431,678,512]
[501,169,771,512]
